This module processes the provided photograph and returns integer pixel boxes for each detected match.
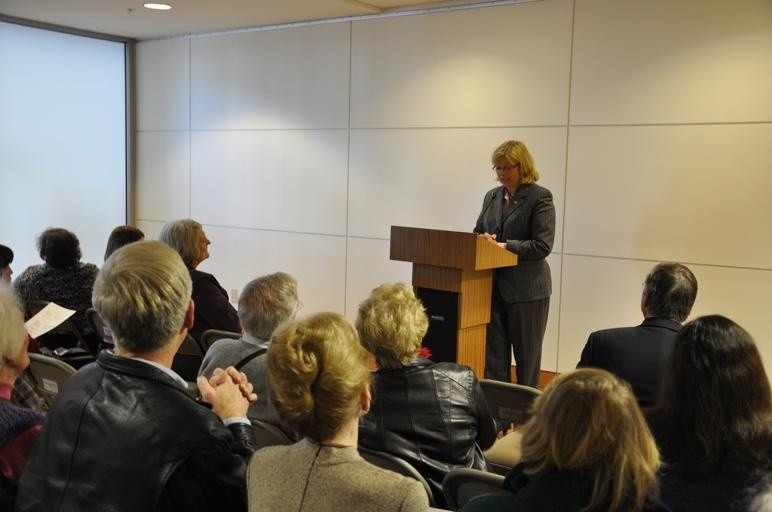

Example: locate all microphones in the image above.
[474,191,496,234]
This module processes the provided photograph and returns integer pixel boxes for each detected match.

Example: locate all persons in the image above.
[576,262,698,409]
[499,368,661,512]
[1,219,495,512]
[642,314,772,512]
[472,140,555,437]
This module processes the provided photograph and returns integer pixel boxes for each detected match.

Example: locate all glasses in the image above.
[494,164,519,172]
